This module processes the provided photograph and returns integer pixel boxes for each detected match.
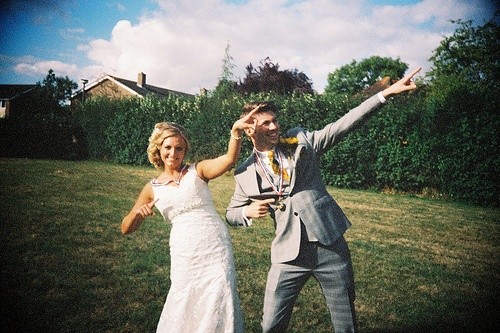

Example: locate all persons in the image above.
[224,66,422,333]
[120,106,260,333]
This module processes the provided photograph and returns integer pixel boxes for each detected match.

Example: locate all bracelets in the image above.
[230,129,244,141]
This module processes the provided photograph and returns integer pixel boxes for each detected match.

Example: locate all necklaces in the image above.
[159,164,187,186]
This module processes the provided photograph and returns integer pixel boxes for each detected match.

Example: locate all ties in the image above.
[268,151,289,183]
